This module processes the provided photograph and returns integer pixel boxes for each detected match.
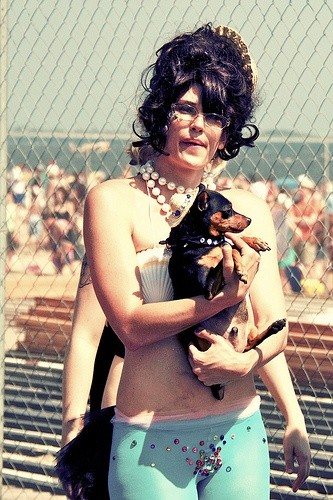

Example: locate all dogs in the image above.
[159,184,288,400]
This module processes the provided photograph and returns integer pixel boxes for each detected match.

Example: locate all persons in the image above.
[2,166,333,500]
[83,23,311,500]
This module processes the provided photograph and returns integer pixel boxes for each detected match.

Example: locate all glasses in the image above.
[171,104,230,131]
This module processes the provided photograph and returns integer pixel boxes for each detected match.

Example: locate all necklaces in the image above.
[138,159,216,225]
